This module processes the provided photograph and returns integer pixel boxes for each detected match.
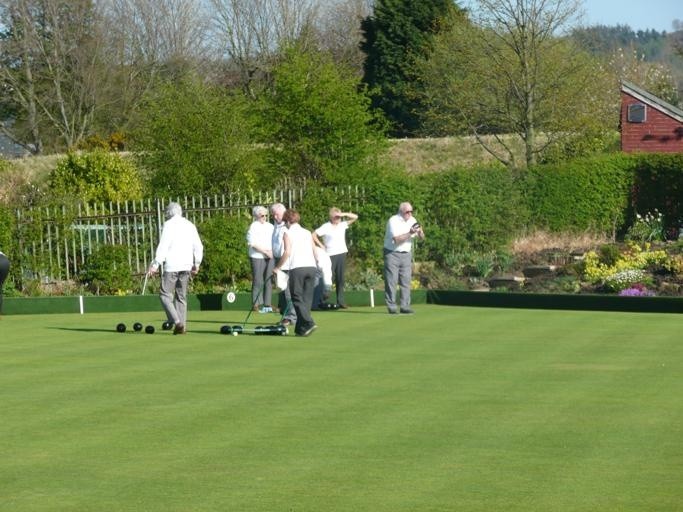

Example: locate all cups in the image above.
[261,214,267,217]
[405,210,412,213]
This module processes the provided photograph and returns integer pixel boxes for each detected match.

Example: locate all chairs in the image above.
[312,303,347,311]
[266,305,280,313]
[173,323,184,334]
[253,306,259,312]
[301,322,317,336]
[281,318,291,327]
[400,308,412,313]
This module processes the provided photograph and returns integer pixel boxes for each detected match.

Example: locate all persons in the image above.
[312,207,360,308]
[383,201,425,315]
[269,203,298,324]
[148,202,204,335]
[273,209,318,337]
[0,251,10,306]
[246,205,273,313]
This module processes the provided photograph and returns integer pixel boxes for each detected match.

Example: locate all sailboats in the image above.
[116,323,173,334]
[319,303,339,310]
[220,326,289,336]
